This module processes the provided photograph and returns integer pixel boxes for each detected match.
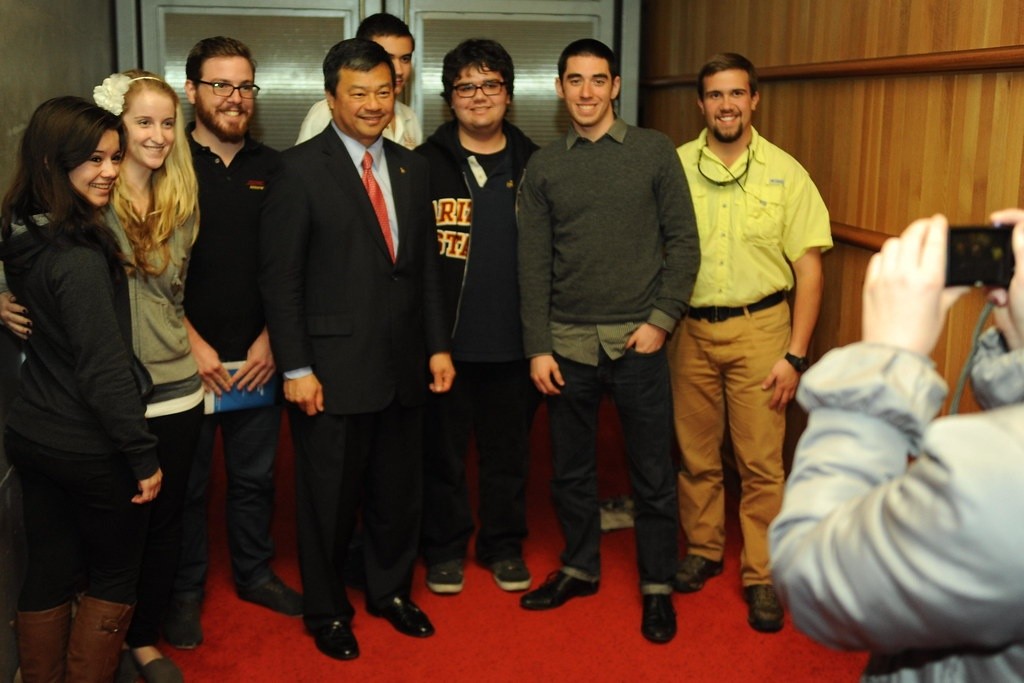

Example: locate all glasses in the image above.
[452,80,505,97]
[189,80,260,99]
[699,146,750,185]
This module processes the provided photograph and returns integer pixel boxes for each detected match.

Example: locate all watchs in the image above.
[784,353,809,372]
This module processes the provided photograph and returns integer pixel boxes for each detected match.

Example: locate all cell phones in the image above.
[945,226,1015,288]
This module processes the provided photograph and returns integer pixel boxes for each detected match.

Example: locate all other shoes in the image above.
[116,650,143,683]
[145,654,184,683]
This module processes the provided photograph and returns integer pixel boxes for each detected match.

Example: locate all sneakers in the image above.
[673,553,724,593]
[745,584,783,634]
[238,570,302,617]
[492,556,530,591]
[426,549,463,594]
[163,592,202,650]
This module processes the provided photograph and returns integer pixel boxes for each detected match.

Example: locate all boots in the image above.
[63,597,134,683]
[17,605,66,683]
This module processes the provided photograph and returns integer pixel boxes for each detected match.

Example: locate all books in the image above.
[204,361,274,416]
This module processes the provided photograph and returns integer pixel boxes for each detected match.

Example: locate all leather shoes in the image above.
[312,620,359,662]
[522,569,598,610]
[367,591,435,638]
[642,585,676,643]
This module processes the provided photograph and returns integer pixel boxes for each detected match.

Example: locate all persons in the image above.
[165,36,304,650]
[0,68,204,683]
[664,55,835,633]
[770,207,1024,683]
[514,39,701,641]
[407,39,545,593]
[258,39,457,659]
[294,11,422,590]
[0,96,162,683]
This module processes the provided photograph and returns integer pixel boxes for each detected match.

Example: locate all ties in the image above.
[360,152,394,264]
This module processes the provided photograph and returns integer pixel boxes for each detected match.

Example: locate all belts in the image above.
[685,289,786,323]
